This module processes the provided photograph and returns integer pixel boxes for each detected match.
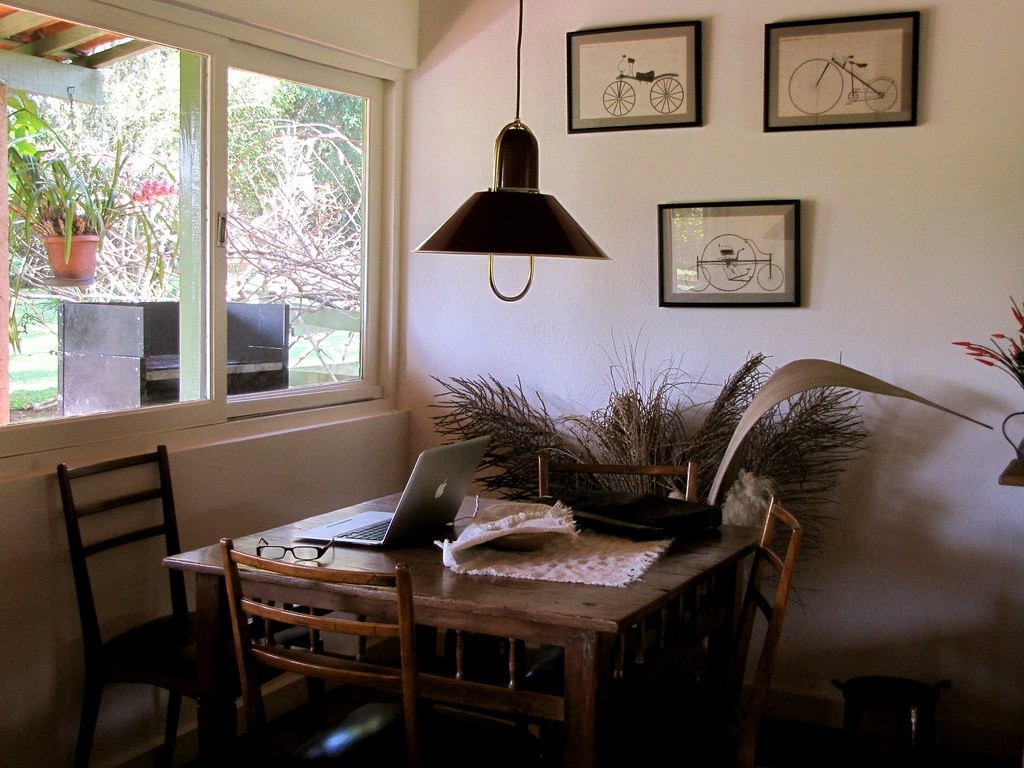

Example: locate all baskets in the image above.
[832,675,952,768]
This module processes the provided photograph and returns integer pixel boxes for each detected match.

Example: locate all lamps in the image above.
[411,0,613,302]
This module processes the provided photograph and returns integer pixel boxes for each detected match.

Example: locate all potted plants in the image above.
[6,91,178,357]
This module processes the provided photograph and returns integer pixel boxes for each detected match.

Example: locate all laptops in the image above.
[294,435,491,547]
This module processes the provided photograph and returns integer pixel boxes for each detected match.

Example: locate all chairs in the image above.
[56,444,325,768]
[219,536,540,768]
[510,455,698,695]
[525,496,803,768]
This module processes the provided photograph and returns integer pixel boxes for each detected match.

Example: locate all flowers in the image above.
[952,295,1024,389]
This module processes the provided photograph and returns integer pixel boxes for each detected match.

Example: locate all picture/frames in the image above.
[565,19,702,134]
[762,10,921,133]
[656,198,803,308]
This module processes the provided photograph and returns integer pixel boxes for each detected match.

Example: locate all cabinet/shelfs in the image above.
[56,295,291,417]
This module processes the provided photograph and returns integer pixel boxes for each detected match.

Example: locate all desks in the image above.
[161,493,761,768]
